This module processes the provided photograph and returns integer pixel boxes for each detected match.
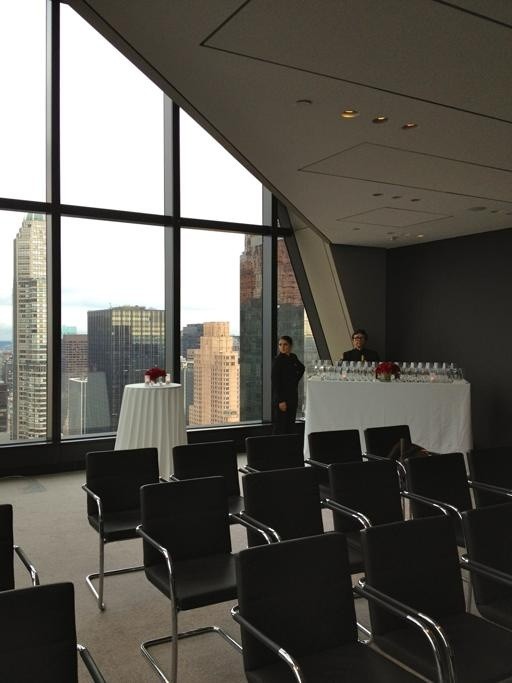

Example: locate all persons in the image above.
[335,330,382,375]
[271,336,306,437]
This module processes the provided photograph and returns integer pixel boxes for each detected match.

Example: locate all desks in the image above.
[302,373,474,463]
[111,381,190,483]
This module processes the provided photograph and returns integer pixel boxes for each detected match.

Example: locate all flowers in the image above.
[374,363,401,381]
[145,367,166,385]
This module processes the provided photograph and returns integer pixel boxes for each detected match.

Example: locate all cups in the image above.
[144,373,171,386]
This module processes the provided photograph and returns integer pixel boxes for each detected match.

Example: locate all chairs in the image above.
[0,578,108,682]
[0,502,39,591]
[83,425,512,682]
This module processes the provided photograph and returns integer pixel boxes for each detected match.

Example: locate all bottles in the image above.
[308,360,462,382]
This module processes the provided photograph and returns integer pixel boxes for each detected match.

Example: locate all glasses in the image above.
[353,336,366,341]
